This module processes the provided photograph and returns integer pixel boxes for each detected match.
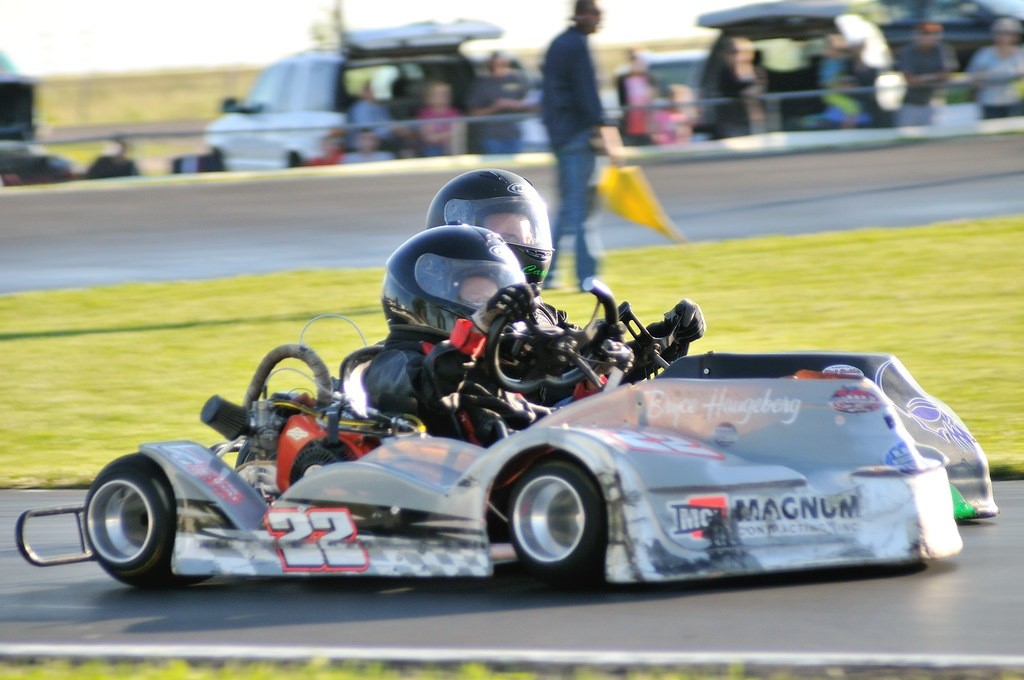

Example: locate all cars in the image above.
[879,0,1024,126]
[0,52,40,142]
[614,0,883,146]
[206,19,503,170]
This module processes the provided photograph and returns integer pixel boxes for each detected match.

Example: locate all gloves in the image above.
[471,283,541,334]
[576,318,635,374]
[660,299,705,361]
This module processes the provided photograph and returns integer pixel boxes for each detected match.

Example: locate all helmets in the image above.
[426,169,555,297]
[381,225,527,366]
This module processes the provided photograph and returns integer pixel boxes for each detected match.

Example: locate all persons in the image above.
[424,168,706,382]
[968,18,1023,118]
[900,24,958,128]
[90,138,137,177]
[313,52,529,166]
[339,223,633,440]
[619,21,906,145]
[540,1,630,292]
